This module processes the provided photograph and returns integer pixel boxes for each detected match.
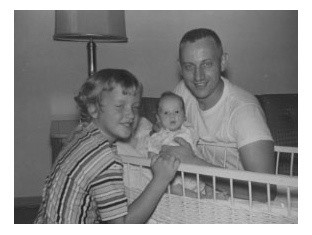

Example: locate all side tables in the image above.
[50,120,79,163]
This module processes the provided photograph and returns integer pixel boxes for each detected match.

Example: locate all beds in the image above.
[120,143,298,224]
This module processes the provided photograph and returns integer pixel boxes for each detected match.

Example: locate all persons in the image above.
[34,68,181,224]
[148,28,277,204]
[148,92,220,195]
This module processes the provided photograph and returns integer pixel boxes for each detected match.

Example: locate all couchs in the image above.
[139,94,299,168]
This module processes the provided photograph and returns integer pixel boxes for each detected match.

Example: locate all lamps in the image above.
[54,10,128,77]
